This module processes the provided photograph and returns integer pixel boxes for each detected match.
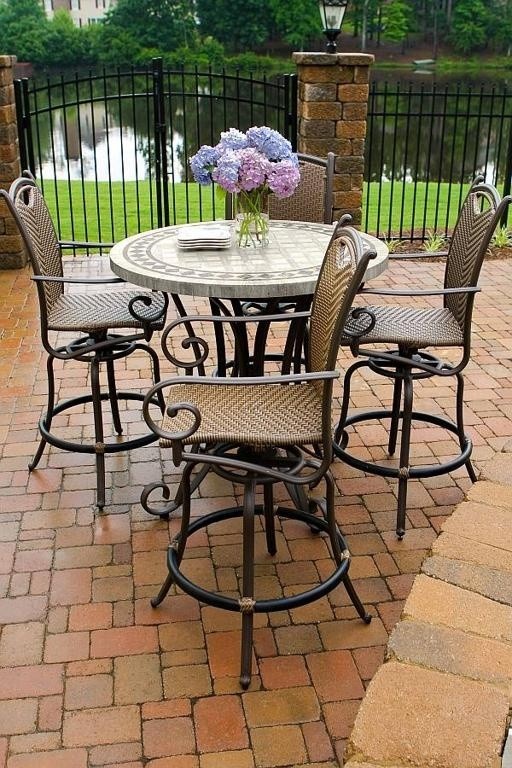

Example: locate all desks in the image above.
[107,216,390,537]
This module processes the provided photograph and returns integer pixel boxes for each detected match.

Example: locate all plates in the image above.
[176,225,231,250]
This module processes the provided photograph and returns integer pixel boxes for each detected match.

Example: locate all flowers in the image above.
[186,120,302,246]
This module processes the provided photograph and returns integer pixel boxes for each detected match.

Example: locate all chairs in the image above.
[223,147,338,380]
[3,166,173,514]
[144,207,376,690]
[327,173,512,547]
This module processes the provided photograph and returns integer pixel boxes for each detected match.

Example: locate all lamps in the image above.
[315,0,350,53]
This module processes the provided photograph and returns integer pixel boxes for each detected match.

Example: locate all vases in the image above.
[232,190,272,250]
